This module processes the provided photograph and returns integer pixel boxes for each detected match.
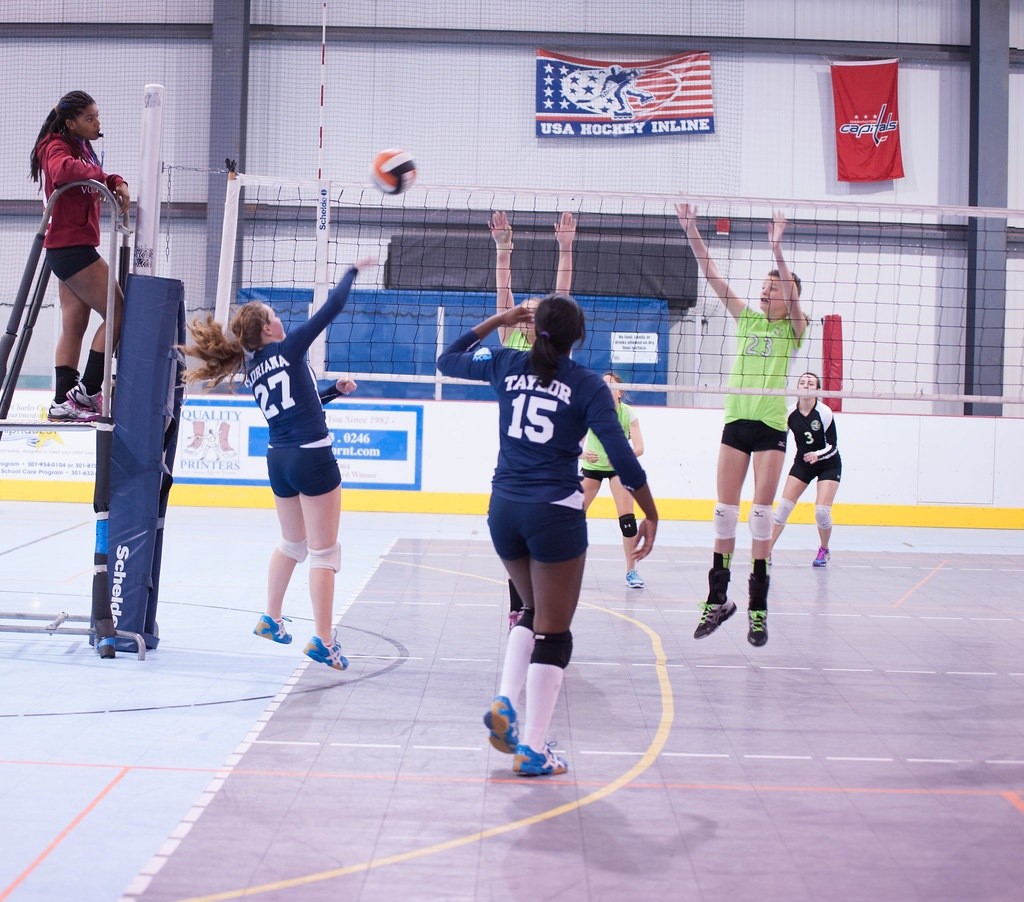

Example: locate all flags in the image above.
[831,58,905,183]
[536,49,714,137]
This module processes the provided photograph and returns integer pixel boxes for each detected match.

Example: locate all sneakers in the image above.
[252,614,294,644]
[303,629,350,671]
[506,611,525,635]
[484,694,520,754]
[66,380,102,418]
[813,545,831,567]
[47,398,101,422]
[513,740,568,776]
[746,609,769,647]
[694,595,737,639]
[625,568,645,588]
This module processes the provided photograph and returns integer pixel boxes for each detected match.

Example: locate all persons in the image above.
[486,209,577,636]
[29,91,130,424]
[580,373,644,588]
[182,258,375,670]
[769,372,842,566]
[673,203,808,648]
[435,293,660,776]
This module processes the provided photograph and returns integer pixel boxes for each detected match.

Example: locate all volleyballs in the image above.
[369,149,417,196]
